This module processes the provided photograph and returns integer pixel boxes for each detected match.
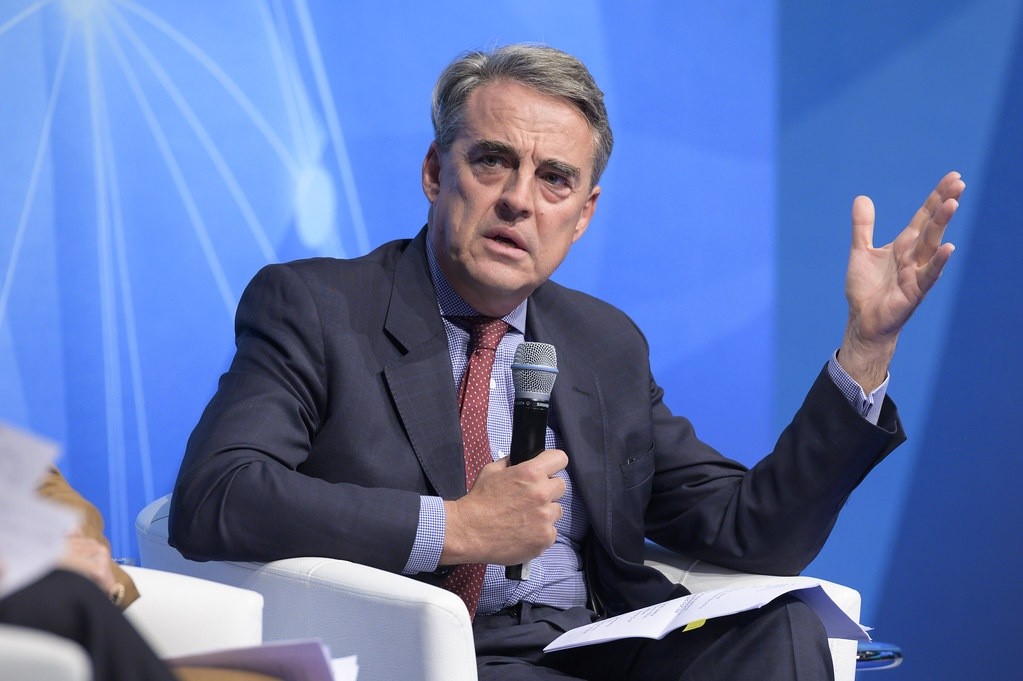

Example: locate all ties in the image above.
[436,316,514,625]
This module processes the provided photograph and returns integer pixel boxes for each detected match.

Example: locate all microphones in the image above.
[504,342,559,582]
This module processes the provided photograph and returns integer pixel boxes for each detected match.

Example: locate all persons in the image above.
[167,43,967,681]
[0,426,178,681]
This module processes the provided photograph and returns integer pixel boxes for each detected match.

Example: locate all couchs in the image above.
[0,562,267,681]
[136,494,861,681]
